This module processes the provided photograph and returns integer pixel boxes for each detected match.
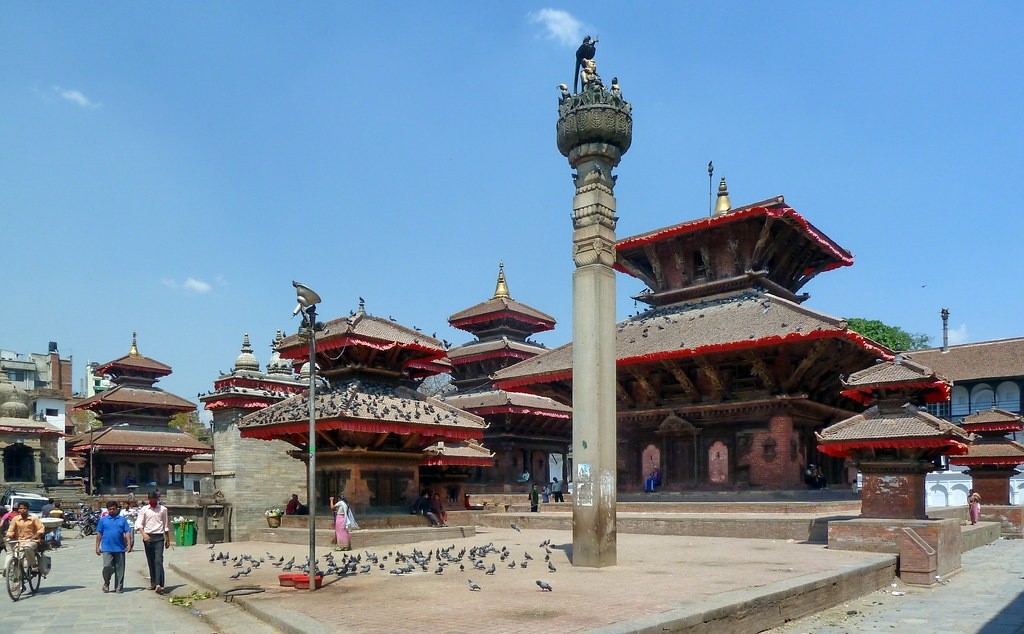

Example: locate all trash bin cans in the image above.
[172,519,195,547]
[265,511,284,528]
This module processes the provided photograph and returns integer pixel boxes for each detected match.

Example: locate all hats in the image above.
[148,489,160,501]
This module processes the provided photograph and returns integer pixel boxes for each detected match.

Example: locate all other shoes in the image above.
[114,590,123,593]
[334,547,349,551]
[31,564,38,571]
[147,585,164,592]
[971,522,976,526]
[12,583,20,591]
[102,582,109,593]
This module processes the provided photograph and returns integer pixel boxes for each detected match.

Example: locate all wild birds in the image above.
[33,386,169,421]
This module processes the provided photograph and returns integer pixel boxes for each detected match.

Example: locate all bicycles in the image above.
[79,505,100,536]
[5,537,42,601]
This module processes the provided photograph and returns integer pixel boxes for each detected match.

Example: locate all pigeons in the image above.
[467,579,481,590]
[535,579,552,591]
[207,523,556,580]
[198,272,824,425]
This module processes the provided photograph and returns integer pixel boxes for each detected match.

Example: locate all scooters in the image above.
[64,510,75,521]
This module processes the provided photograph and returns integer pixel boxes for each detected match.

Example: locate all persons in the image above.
[530,483,539,511]
[0,498,66,595]
[805,462,829,490]
[644,465,662,494]
[329,493,352,551]
[966,489,981,525]
[523,470,530,482]
[555,58,623,103]
[541,485,550,504]
[95,485,170,593]
[412,490,448,528]
[552,477,565,502]
[286,494,308,515]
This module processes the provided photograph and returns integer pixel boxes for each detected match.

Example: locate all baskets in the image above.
[266,515,281,528]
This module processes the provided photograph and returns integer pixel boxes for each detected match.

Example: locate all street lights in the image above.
[291,282,327,592]
[90,424,131,496]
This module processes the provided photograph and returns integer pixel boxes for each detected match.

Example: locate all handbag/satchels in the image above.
[528,490,535,500]
[39,550,52,577]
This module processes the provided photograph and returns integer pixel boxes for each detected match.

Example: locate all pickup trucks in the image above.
[0,492,49,518]
